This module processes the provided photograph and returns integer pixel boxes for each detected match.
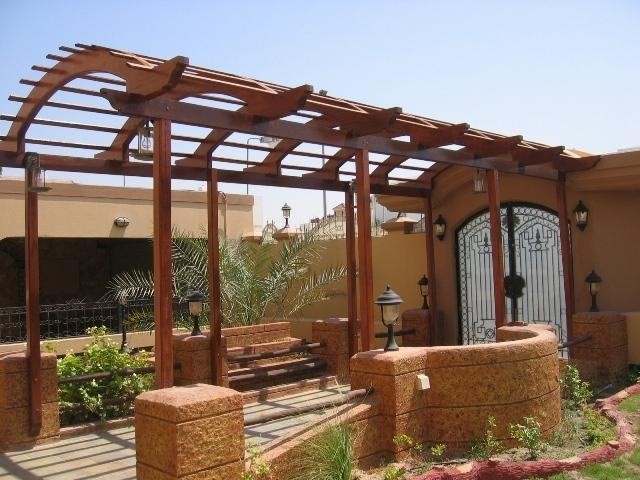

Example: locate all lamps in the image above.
[432,213,447,242]
[373,282,404,350]
[472,168,486,192]
[583,269,604,312]
[26,152,52,194]
[186,291,205,336]
[281,201,292,228]
[128,120,153,161]
[114,217,130,227]
[417,274,429,309]
[572,200,589,232]
